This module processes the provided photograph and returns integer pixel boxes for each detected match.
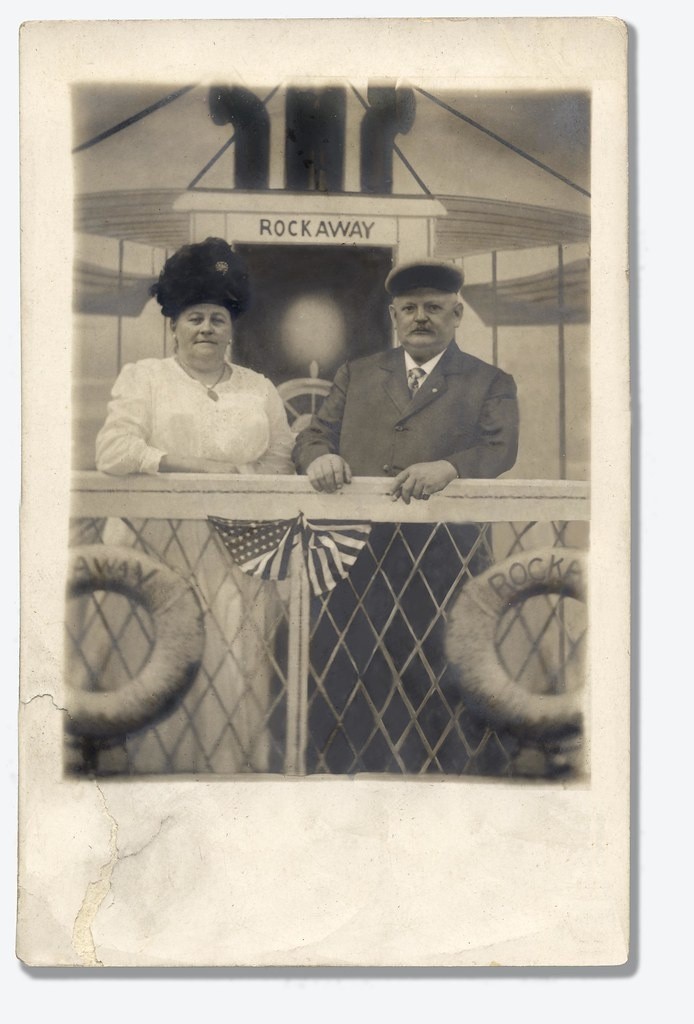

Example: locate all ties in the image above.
[407,368,426,400]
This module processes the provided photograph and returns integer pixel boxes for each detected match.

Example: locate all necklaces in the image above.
[176,356,226,402]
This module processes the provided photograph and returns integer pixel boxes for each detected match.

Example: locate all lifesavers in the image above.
[63,545,206,738]
[442,549,587,731]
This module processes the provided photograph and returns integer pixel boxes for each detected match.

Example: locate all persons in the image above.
[76,235,292,774]
[293,259,520,773]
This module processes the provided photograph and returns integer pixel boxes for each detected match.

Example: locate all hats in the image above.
[152,237,251,321]
[384,259,465,294]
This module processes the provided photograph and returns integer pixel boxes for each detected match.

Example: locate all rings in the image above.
[422,493,430,500]
